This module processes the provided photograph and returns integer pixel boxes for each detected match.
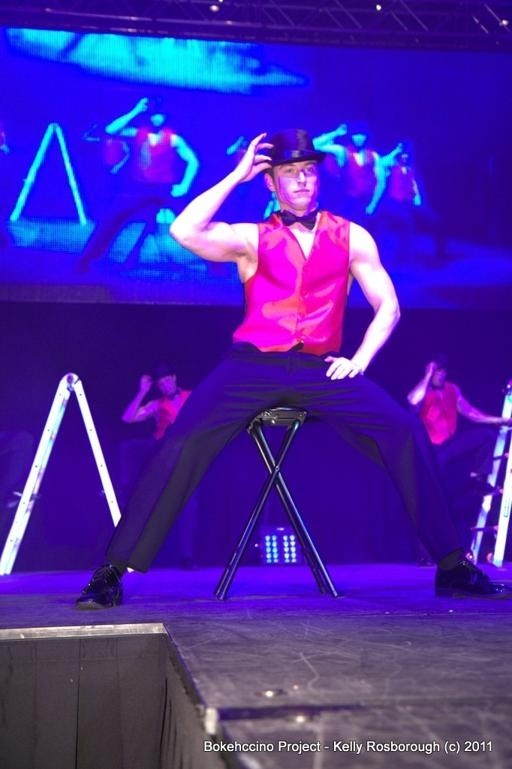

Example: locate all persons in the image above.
[74,94,230,284]
[405,357,511,498]
[227,135,251,193]
[378,140,455,261]
[83,124,132,175]
[119,366,192,442]
[72,123,512,616]
[310,125,413,267]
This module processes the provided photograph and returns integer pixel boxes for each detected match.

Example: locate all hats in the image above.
[151,363,179,383]
[252,126,328,168]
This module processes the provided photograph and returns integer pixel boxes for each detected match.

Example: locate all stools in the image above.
[213,402,339,601]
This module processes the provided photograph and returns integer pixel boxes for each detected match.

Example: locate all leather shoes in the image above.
[74,563,124,613]
[432,556,512,601]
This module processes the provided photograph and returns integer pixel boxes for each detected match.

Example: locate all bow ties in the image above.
[273,206,319,232]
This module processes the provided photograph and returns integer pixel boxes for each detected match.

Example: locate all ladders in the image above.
[468,393,512,568]
[0,373,135,575]
